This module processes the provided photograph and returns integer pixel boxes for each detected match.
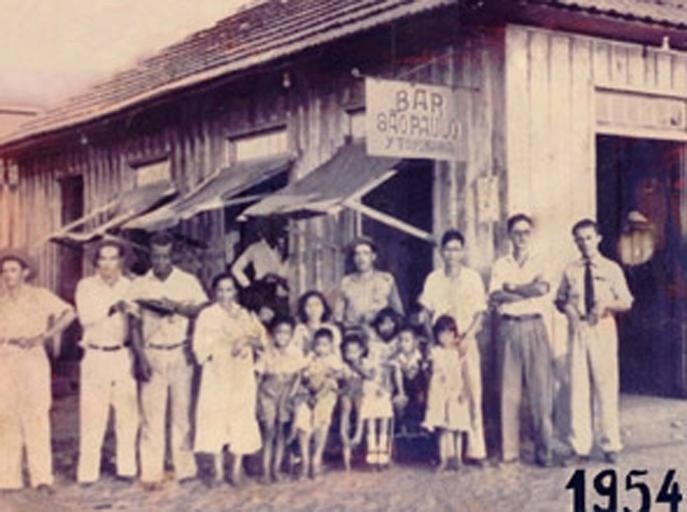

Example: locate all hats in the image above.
[1,247,41,283]
[347,235,382,253]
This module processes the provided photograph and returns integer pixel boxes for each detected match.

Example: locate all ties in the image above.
[582,260,593,314]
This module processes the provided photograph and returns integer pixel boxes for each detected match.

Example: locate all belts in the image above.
[145,341,190,350]
[577,310,611,322]
[88,340,131,354]
[502,310,541,320]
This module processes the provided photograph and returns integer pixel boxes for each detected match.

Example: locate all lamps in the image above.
[613,210,658,267]
